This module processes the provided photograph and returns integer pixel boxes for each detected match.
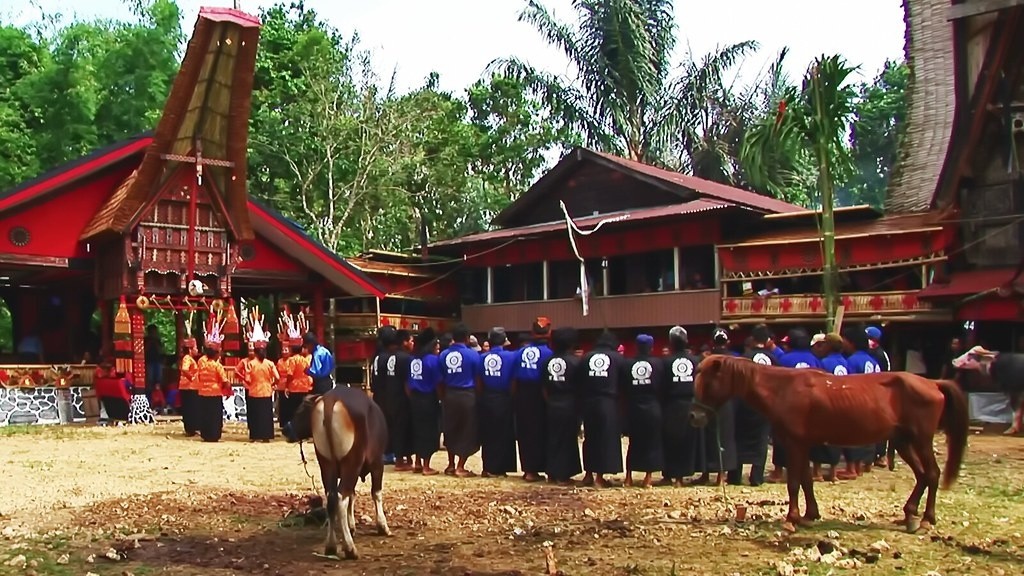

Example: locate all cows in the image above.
[283,384,393,560]
[952,345,1024,437]
[686,353,970,535]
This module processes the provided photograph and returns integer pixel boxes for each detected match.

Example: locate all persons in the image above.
[372,317,891,489]
[179,337,232,441]
[938,337,970,390]
[682,269,711,290]
[575,268,602,296]
[235,333,335,444]
[745,279,779,299]
[144,324,167,389]
[82,350,94,364]
[18,330,44,364]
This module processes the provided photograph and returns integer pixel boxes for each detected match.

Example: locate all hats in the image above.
[865,326,883,343]
[202,305,228,352]
[531,316,552,339]
[181,310,197,348]
[469,335,482,352]
[636,334,655,349]
[810,333,827,347]
[416,326,436,347]
[711,327,731,346]
[487,326,508,347]
[241,305,310,349]
